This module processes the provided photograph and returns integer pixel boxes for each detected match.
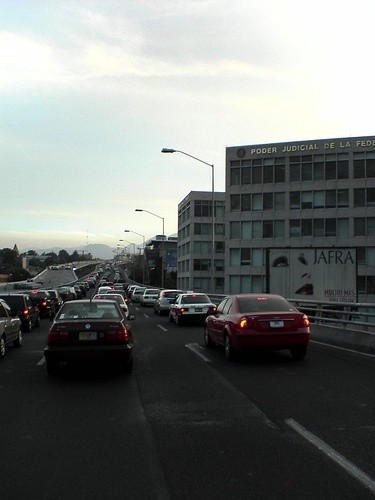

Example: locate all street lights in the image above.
[161,147,215,293]
[124,229,146,283]
[119,239,136,282]
[135,208,165,288]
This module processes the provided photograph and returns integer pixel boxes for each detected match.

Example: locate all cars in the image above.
[127,284,217,326]
[92,261,123,285]
[43,282,136,375]
[49,260,72,270]
[0,272,98,359]
[204,293,312,362]
